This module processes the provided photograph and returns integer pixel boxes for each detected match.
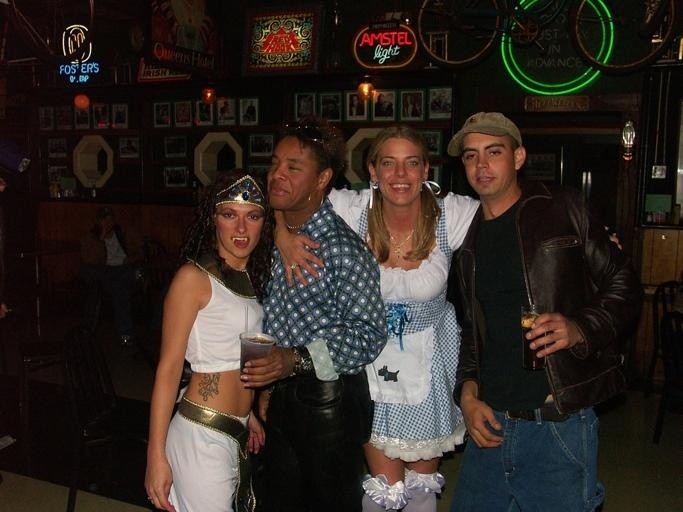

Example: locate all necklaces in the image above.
[283,220,304,229]
[383,220,416,263]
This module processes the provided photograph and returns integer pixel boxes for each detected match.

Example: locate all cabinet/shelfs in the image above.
[630,62,682,382]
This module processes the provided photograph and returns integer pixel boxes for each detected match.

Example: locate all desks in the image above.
[0,242,81,355]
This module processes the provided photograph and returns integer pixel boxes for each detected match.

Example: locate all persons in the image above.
[199,102,211,121]
[142,166,273,512]
[219,101,233,120]
[236,116,389,511]
[95,105,107,120]
[242,100,256,120]
[375,93,393,118]
[114,106,127,120]
[322,99,339,119]
[156,104,170,120]
[403,93,422,118]
[349,94,364,116]
[269,123,622,511]
[176,103,190,120]
[441,110,646,511]
[297,95,313,116]
[431,89,450,113]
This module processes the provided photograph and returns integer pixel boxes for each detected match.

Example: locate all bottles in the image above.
[672,204,680,226]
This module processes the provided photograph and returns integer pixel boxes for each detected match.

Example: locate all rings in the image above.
[146,496,151,502]
[289,264,298,271]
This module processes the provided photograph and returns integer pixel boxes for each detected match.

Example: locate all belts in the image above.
[507,402,570,422]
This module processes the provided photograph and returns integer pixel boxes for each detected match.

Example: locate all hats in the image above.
[448,112,522,156]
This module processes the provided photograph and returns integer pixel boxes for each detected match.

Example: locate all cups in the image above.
[238,331,276,383]
[520,302,545,372]
[646,212,666,224]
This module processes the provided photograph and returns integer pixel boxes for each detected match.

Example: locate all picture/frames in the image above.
[422,131,446,155]
[117,136,142,159]
[247,134,279,160]
[165,166,191,189]
[245,166,279,185]
[164,133,191,159]
[44,141,81,191]
[429,165,445,187]
[293,88,455,122]
[42,102,131,130]
[152,99,259,127]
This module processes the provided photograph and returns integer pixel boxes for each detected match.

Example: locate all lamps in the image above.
[620,119,637,164]
[358,72,375,97]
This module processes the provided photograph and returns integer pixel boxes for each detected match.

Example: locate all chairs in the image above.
[140,242,174,317]
[46,250,83,313]
[58,337,162,512]
[645,281,683,398]
[654,311,682,444]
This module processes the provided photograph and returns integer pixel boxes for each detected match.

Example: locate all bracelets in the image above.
[288,347,301,377]
